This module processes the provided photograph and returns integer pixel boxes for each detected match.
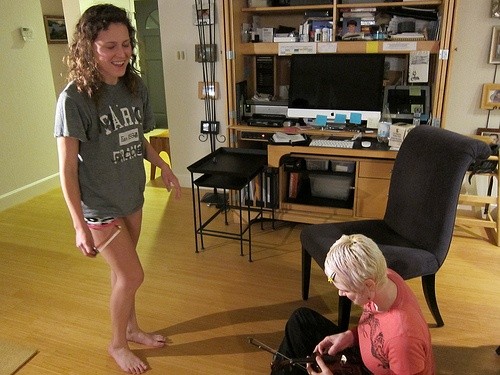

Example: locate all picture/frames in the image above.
[192,5,215,25]
[197,82,218,99]
[488,25,500,64]
[477,128,500,161]
[44,15,68,44]
[195,44,217,62]
[480,83,500,110]
[201,121,219,134]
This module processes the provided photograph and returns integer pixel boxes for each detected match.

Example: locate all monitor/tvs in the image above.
[383,84,431,121]
[287,52,385,121]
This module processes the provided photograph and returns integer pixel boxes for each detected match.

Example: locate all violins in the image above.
[269,348,368,375]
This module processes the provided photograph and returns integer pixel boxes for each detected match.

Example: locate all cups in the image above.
[249,32,259,43]
[373,31,387,40]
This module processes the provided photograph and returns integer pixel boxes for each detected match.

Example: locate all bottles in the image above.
[377,103,392,142]
[413,113,421,126]
[315,29,322,42]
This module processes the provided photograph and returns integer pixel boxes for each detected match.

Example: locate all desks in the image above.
[186,146,275,263]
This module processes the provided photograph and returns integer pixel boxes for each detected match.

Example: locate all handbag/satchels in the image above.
[468,142,499,170]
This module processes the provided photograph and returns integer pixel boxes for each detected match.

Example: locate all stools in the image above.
[453,135,500,246]
[149,129,173,180]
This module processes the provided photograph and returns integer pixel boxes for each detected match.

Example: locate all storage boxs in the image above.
[304,158,355,199]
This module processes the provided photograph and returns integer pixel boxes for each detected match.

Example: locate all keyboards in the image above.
[308,138,355,150]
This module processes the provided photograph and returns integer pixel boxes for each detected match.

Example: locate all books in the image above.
[272,131,305,142]
[336,7,376,41]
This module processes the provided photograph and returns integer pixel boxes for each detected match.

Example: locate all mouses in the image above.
[361,140,372,147]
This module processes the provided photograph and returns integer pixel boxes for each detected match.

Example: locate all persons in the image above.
[269,233,437,375]
[55,4,180,375]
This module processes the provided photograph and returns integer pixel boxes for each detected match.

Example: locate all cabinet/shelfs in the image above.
[223,0,460,224]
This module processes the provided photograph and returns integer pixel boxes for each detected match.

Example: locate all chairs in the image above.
[300,126,492,333]
